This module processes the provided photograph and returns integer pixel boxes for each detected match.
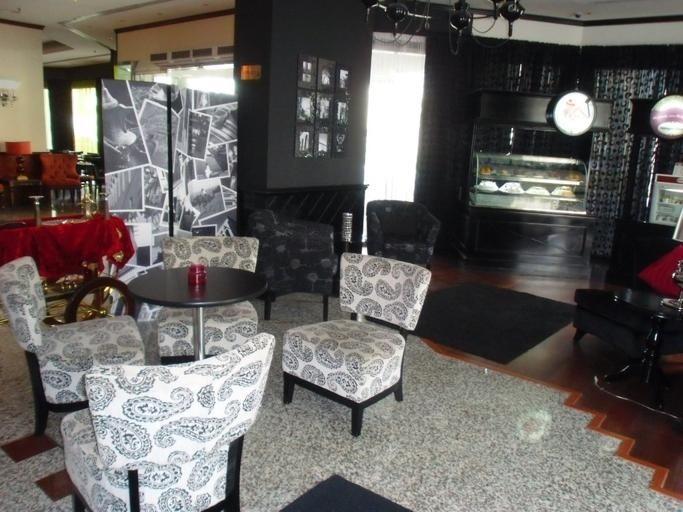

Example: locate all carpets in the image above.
[278,473,414,511]
[364,280,580,366]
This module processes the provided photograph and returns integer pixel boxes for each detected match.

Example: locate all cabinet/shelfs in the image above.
[469,152,589,216]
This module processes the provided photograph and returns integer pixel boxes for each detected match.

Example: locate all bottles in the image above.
[187,263,207,285]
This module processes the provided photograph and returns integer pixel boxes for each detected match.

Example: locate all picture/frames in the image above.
[292,53,354,164]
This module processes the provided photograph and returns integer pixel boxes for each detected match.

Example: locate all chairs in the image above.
[248,208,339,321]
[279,249,433,437]
[366,199,442,271]
[60,333,278,511]
[1,141,101,209]
[0,254,148,439]
[604,219,683,293]
[157,230,259,363]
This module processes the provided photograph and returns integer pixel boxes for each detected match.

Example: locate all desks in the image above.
[125,263,268,360]
[573,288,683,380]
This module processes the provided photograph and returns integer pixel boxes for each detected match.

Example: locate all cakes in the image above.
[479,180,573,197]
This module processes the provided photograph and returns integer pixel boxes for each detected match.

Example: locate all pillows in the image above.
[639,244,683,298]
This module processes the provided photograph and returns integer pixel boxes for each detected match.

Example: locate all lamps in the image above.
[366,0,525,57]
